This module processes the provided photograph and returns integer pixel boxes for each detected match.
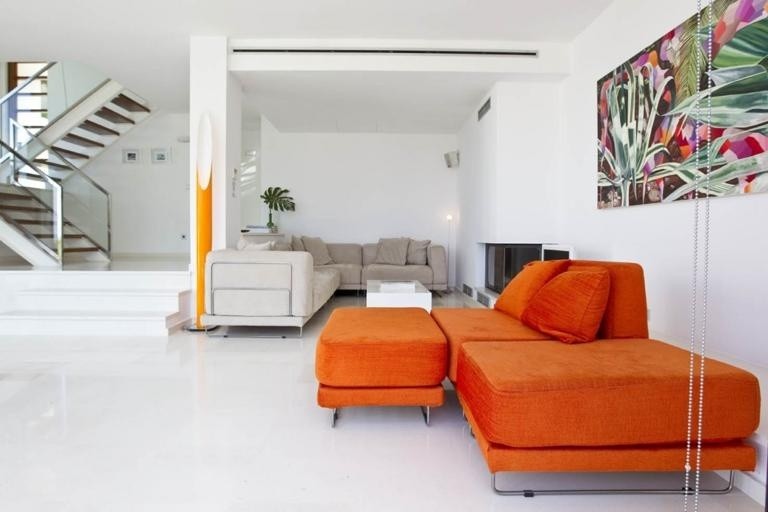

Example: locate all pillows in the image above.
[407,239,431,265]
[270,235,334,265]
[494,259,571,320]
[494,259,610,344]
[375,237,410,266]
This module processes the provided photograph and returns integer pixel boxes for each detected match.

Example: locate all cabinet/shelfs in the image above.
[459,281,499,309]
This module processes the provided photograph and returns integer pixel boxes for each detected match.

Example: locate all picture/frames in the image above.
[123,146,171,164]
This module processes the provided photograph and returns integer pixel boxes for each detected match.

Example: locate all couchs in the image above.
[200,243,448,338]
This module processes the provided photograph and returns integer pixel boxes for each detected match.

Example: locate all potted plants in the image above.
[260,187,295,228]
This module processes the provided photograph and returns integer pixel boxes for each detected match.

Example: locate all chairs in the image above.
[430,261,648,388]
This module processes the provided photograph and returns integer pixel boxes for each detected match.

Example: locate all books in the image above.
[240,225,269,233]
[382,281,415,287]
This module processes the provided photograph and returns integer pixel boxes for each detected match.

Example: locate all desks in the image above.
[242,233,284,243]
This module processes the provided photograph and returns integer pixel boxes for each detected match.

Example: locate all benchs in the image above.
[456,338,760,496]
[315,306,448,428]
